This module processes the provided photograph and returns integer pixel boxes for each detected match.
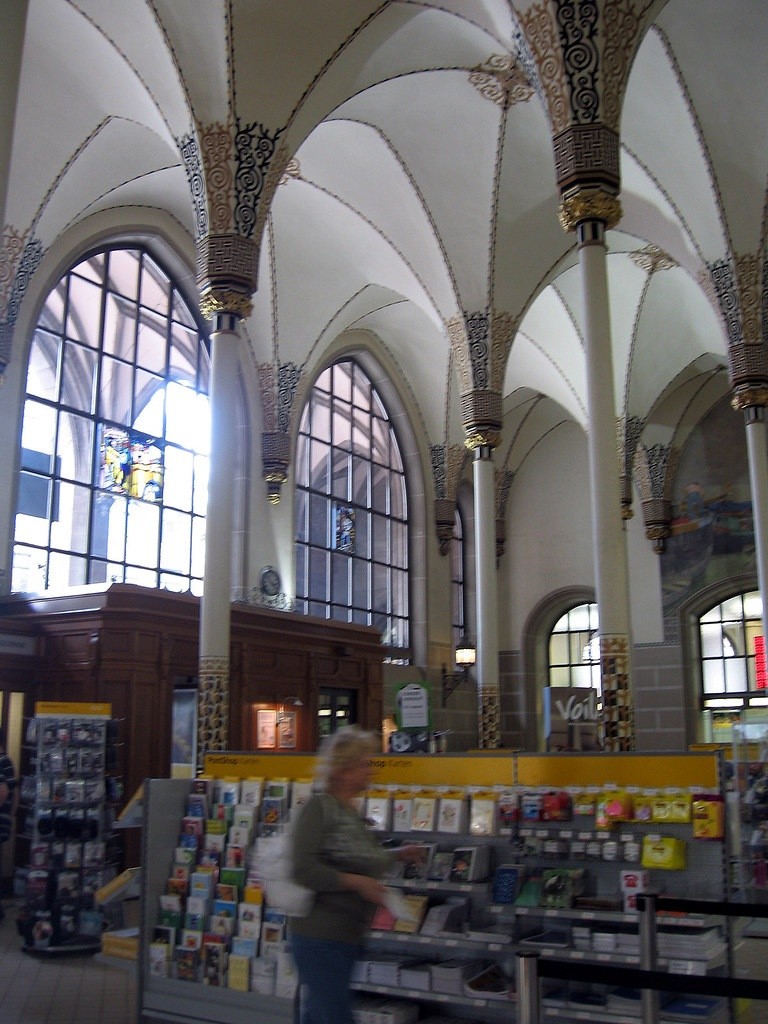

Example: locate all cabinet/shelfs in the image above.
[12,715,124,955]
[95,740,768,1024]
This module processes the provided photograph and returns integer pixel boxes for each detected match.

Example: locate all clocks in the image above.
[262,569,281,595]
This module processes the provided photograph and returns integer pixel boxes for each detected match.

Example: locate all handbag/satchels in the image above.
[257,791,342,920]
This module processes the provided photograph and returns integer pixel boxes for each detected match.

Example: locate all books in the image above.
[145,774,518,1002]
[526,921,727,1019]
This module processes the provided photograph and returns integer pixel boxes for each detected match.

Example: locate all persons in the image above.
[285,729,429,1024]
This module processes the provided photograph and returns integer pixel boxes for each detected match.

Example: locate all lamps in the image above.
[441,636,476,708]
